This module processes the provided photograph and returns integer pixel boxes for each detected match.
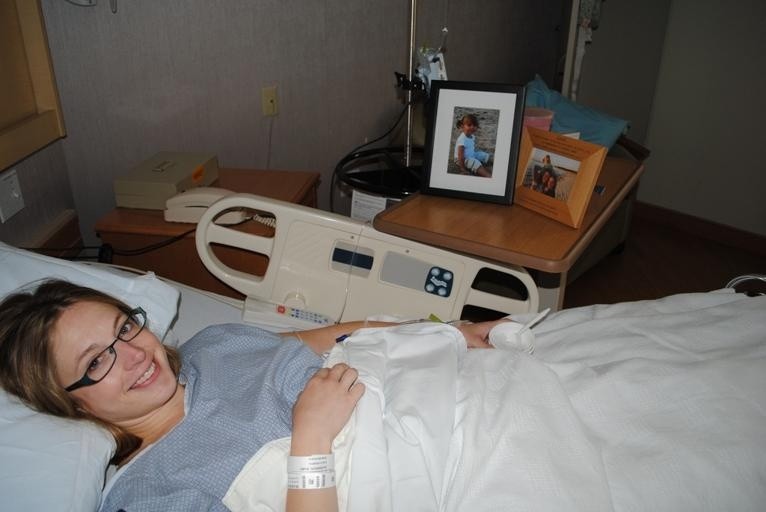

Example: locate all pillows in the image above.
[0,236,183,512]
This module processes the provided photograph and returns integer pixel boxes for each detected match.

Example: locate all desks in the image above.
[372,139,646,339]
[95,165,320,299]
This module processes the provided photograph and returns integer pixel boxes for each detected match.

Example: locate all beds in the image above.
[0,200,765,512]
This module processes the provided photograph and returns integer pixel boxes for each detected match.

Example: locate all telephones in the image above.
[164,188,247,225]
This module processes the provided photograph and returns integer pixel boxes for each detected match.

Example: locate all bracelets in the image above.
[286,452,338,491]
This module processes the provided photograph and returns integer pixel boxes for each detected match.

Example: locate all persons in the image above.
[530,155,557,197]
[0,278,518,512]
[454,114,495,179]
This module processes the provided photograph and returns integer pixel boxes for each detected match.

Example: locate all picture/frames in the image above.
[420,79,608,231]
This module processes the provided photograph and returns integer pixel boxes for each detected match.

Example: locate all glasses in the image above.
[65,306,147,393]
